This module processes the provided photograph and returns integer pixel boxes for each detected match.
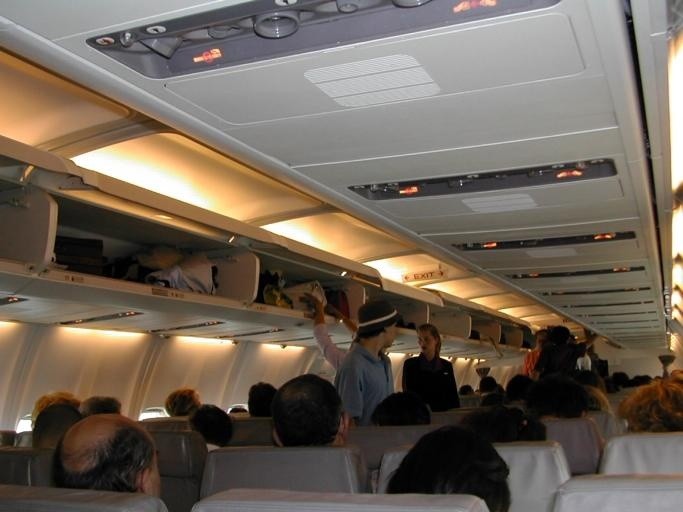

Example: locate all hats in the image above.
[357,300,402,337]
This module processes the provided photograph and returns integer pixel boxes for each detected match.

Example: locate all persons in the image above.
[166,389,201,417]
[32,391,79,428]
[271,373,349,446]
[33,405,81,448]
[402,323,461,412]
[370,392,431,426]
[79,395,122,416]
[460,325,683,443]
[248,382,277,418]
[186,403,233,451]
[298,291,358,366]
[51,413,162,498]
[230,408,247,413]
[333,299,403,425]
[387,424,511,511]
